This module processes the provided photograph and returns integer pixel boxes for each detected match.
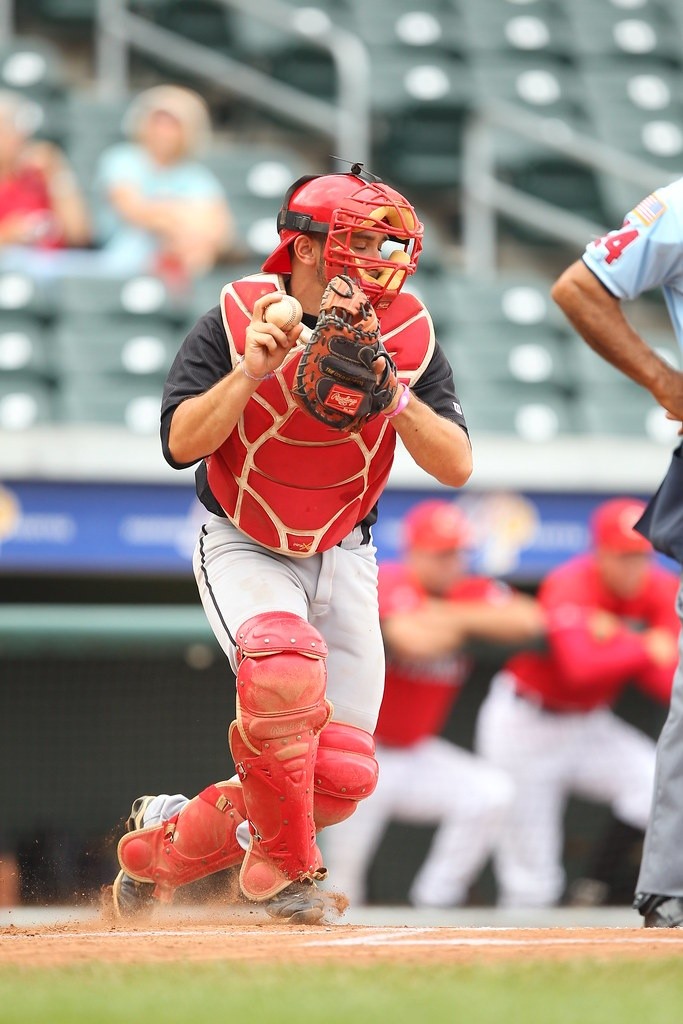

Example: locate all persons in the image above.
[112,154,474,925]
[316,499,544,910]
[93,86,232,275]
[1,86,87,250]
[556,179,683,927]
[474,497,681,907]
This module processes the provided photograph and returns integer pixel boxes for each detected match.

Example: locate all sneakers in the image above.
[265,879,326,923]
[116,793,188,922]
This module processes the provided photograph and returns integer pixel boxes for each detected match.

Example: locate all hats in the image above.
[405,496,472,555]
[590,496,656,555]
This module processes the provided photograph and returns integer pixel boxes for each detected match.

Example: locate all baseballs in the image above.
[264,294,303,332]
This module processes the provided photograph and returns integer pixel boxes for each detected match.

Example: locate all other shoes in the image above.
[645,897,682,927]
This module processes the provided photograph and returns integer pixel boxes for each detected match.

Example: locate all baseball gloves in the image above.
[291,275,397,433]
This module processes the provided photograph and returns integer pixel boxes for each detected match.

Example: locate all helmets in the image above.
[261,175,390,276]
